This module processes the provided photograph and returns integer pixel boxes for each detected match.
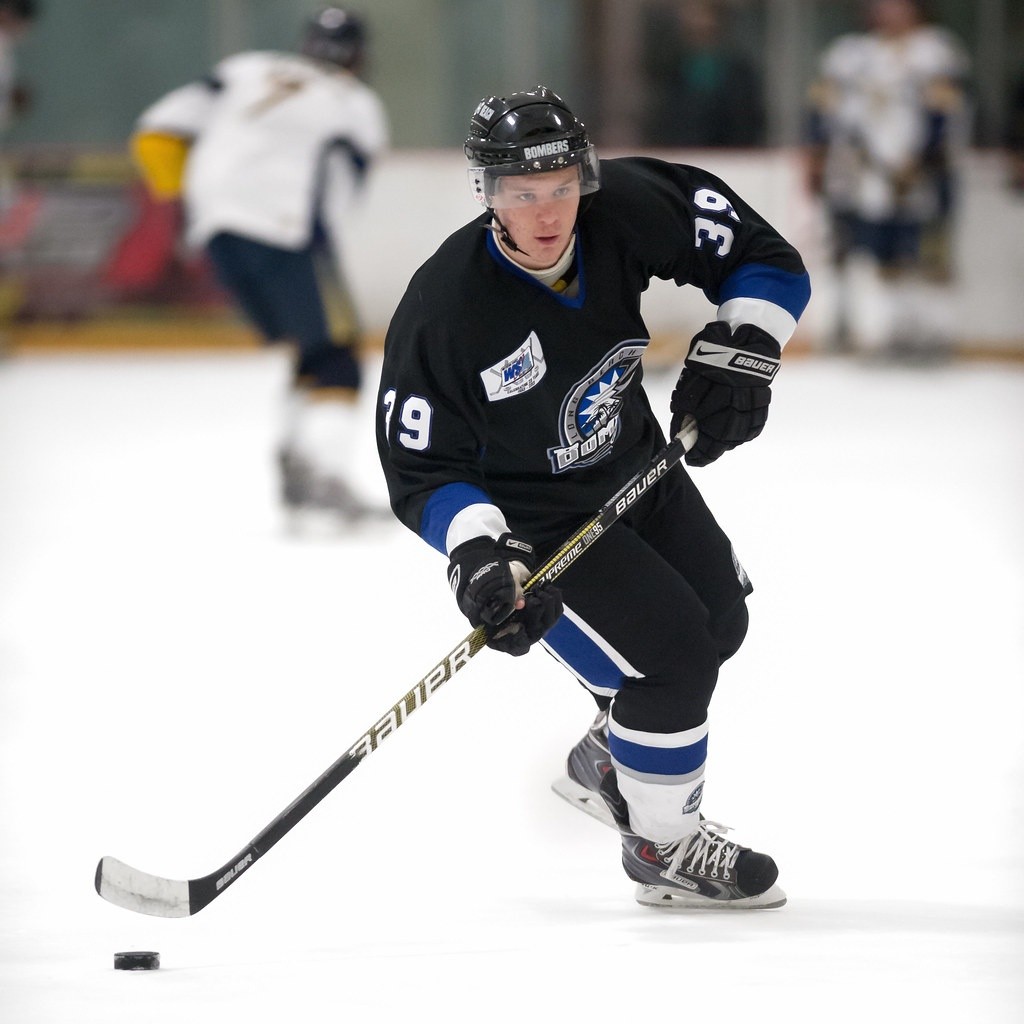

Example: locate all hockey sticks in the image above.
[89,413,699,921]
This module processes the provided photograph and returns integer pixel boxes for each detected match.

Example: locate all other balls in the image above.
[111,951,161,971]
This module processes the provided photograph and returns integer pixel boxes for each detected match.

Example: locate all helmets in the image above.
[464,83,604,208]
[301,7,375,51]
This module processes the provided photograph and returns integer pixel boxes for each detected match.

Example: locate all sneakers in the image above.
[551,706,619,832]
[601,765,789,911]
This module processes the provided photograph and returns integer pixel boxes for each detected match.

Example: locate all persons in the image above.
[99,1,391,516]
[376,85,811,909]
[636,0,772,148]
[810,0,971,349]
